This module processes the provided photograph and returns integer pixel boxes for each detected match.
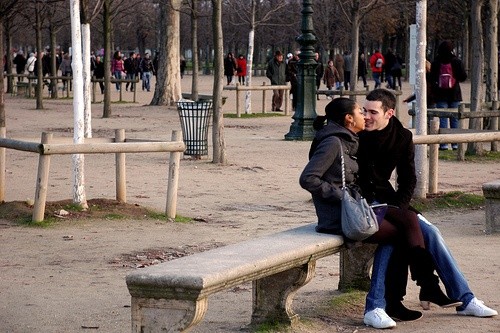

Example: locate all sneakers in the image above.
[364,307,396,329]
[457,297,498,317]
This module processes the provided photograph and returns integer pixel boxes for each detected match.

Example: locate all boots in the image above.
[386,246,422,320]
[409,247,463,310]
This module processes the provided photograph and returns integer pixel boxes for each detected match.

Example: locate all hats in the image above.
[287,53,293,58]
[275,51,283,56]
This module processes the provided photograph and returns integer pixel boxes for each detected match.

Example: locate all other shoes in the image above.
[438,146,448,151]
[453,147,458,151]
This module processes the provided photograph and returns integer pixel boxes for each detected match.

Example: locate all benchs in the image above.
[125,223,379,333]
[182,91,228,107]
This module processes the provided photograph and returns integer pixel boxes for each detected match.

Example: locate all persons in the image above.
[324,61,340,99]
[339,88,499,329]
[112,50,159,92]
[266,51,288,112]
[27,49,72,93]
[224,52,237,85]
[371,46,403,90]
[91,47,105,94]
[3,50,8,78]
[336,50,353,99]
[299,98,464,319]
[314,52,323,99]
[404,41,467,150]
[359,52,369,87]
[287,50,304,111]
[236,54,247,85]
[14,50,26,81]
[180,54,187,78]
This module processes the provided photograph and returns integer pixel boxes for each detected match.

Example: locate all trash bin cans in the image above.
[176,100,213,156]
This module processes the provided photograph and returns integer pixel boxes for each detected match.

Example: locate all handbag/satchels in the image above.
[237,66,243,72]
[341,191,379,241]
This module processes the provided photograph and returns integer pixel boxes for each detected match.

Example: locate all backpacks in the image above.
[439,62,456,88]
[373,54,383,68]
